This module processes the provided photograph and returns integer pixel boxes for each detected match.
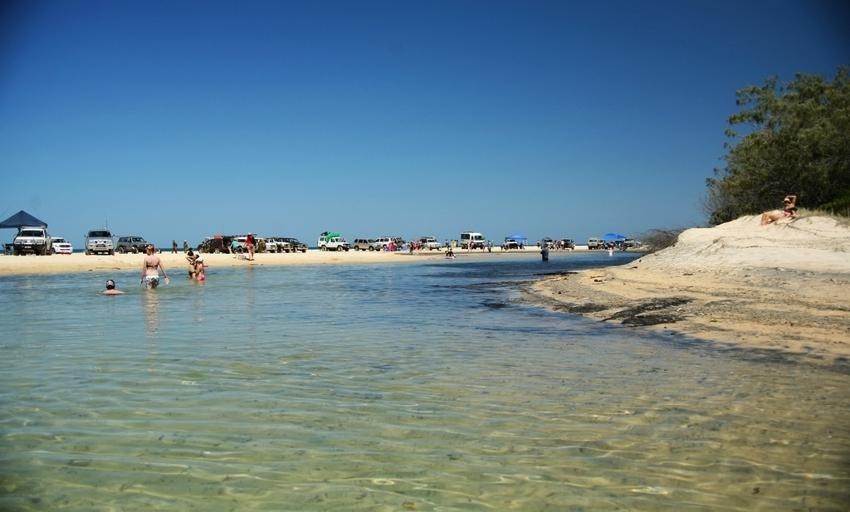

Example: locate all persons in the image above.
[387,237,422,252]
[518,238,627,264]
[138,244,169,291]
[102,280,126,294]
[760,206,790,226]
[446,244,452,258]
[784,195,800,219]
[470,239,493,253]
[172,232,256,282]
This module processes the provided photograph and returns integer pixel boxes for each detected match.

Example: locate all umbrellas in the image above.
[0,210,47,234]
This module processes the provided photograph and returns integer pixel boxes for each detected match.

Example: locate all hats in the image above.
[784,198,791,203]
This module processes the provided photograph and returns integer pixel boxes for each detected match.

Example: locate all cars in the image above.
[541,237,575,250]
[13,226,154,256]
[588,238,642,250]
[502,239,518,250]
[197,236,308,253]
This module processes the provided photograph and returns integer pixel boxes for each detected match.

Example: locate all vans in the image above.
[461,233,485,249]
[317,236,441,252]
